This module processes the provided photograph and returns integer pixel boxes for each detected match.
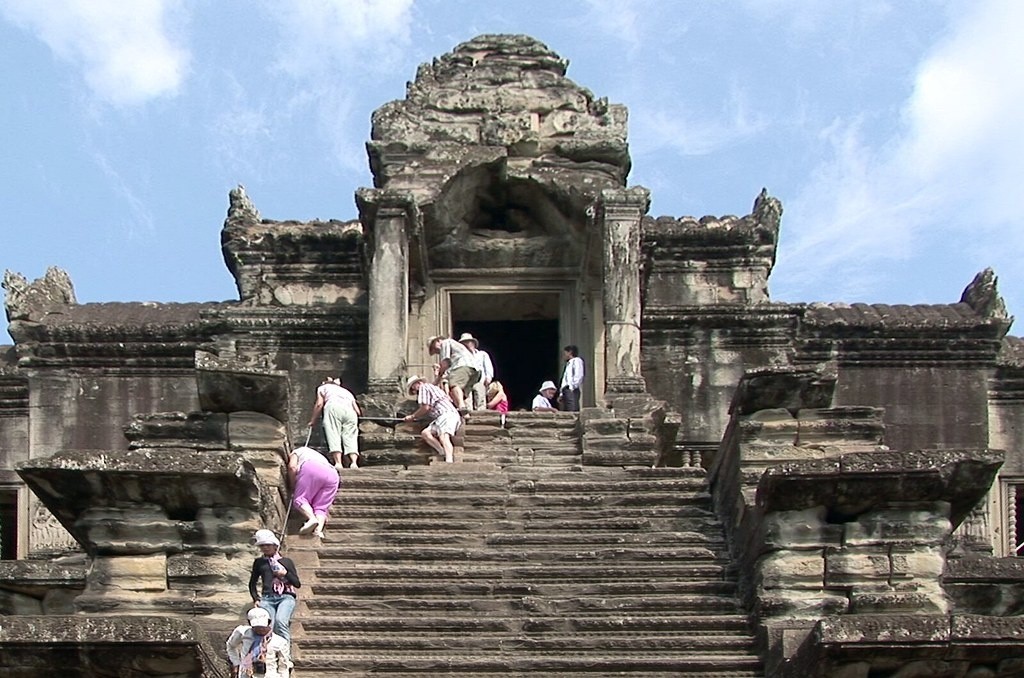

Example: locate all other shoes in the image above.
[334,462,343,468]
[458,406,470,416]
[350,463,359,469]
[300,518,319,534]
[313,530,324,538]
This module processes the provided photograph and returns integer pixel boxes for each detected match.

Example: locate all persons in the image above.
[488,381,507,413]
[459,333,493,411]
[227,608,290,678]
[308,378,360,469]
[558,345,584,412]
[427,335,482,419]
[404,375,460,463]
[531,380,558,413]
[249,529,300,641]
[288,447,340,537]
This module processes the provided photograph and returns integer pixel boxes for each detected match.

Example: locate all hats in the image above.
[539,381,556,393]
[407,375,427,397]
[255,529,280,546]
[247,607,271,627]
[426,335,447,356]
[459,333,479,348]
